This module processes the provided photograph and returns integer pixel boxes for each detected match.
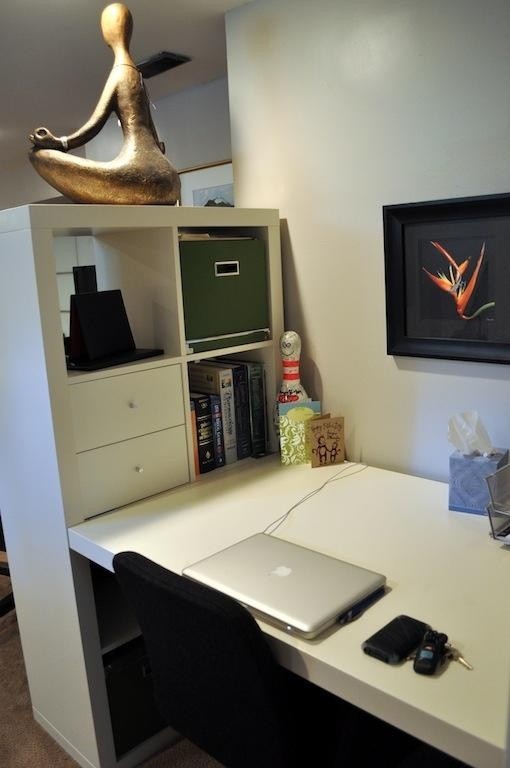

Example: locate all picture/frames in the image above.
[382,193,509,365]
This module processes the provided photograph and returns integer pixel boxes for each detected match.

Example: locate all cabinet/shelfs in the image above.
[0,205,284,768]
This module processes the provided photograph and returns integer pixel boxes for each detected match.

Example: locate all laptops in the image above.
[178,532,389,640]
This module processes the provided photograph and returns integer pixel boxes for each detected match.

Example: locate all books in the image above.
[190,360,266,477]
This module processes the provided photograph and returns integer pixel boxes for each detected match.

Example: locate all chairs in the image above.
[111,552,345,766]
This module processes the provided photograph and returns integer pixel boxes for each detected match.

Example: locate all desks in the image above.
[68,455,509,768]
